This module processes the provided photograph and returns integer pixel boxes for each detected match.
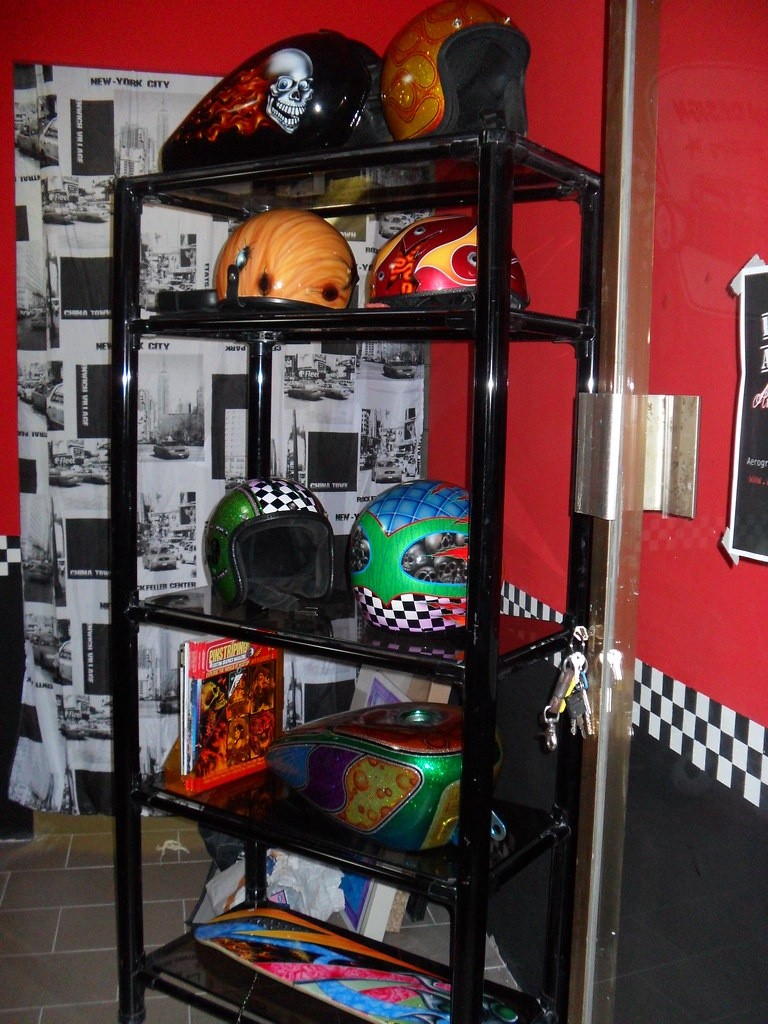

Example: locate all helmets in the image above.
[204,476,335,609]
[349,480,471,637]
[216,207,359,312]
[370,214,531,312]
[380,0,532,143]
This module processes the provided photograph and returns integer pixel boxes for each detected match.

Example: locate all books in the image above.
[177,634,283,793]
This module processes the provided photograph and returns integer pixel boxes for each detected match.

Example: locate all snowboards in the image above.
[194,907,518,1024]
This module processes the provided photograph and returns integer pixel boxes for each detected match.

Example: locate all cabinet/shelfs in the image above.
[112,127,603,1024]
